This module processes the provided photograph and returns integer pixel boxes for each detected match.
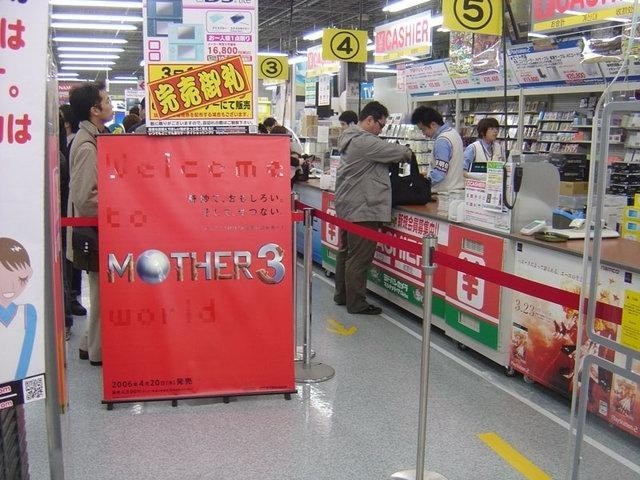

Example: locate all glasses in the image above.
[378,120,385,128]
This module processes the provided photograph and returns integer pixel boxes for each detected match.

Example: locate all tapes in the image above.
[561,207,574,214]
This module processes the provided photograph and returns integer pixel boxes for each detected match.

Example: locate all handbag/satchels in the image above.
[72,225,100,273]
[389,151,432,206]
[295,161,310,182]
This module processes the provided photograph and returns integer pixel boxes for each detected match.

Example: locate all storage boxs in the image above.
[300,115,343,192]
[558,181,639,243]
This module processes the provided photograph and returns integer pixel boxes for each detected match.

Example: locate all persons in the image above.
[333,99,413,316]
[410,106,464,193]
[339,110,358,130]
[55,77,147,367]
[462,117,506,174]
[264,117,304,151]
[257,124,268,134]
[271,127,306,190]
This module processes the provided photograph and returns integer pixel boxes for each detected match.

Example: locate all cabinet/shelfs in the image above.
[375,34,640,180]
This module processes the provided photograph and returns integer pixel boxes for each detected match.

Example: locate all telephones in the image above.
[569,218,606,231]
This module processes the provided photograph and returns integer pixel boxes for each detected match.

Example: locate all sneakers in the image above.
[64,329,73,342]
[68,301,87,316]
[79,348,102,367]
[351,304,383,315]
